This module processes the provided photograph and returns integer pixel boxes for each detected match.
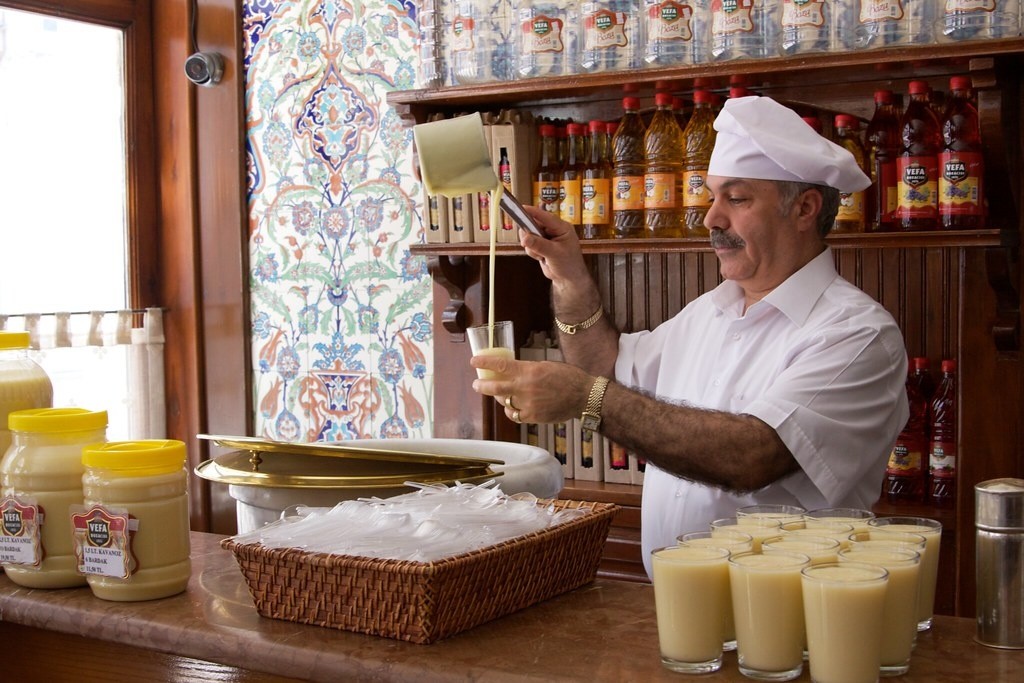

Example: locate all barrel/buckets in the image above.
[230,428,562,540]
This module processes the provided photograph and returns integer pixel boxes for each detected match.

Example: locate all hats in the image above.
[706,96,872,194]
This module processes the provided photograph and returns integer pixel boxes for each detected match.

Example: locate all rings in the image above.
[505,395,515,411]
[513,410,522,423]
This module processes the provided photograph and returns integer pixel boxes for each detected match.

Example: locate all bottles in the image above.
[1,328,54,487]
[77,439,190,602]
[1,403,111,590]
[878,356,959,506]
[409,0,1024,242]
[520,332,646,475]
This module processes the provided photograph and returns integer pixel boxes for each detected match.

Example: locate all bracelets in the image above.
[556,304,604,336]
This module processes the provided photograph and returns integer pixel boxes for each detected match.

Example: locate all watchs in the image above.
[580,376,609,434]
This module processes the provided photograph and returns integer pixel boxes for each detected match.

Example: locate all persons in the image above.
[471,96,910,588]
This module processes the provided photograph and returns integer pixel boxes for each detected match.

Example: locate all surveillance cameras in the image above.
[184,51,225,88]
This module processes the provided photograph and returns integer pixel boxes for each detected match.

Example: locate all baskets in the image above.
[220,492,623,646]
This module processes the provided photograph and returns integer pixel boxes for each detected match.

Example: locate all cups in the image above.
[649,505,942,682]
[465,321,517,388]
